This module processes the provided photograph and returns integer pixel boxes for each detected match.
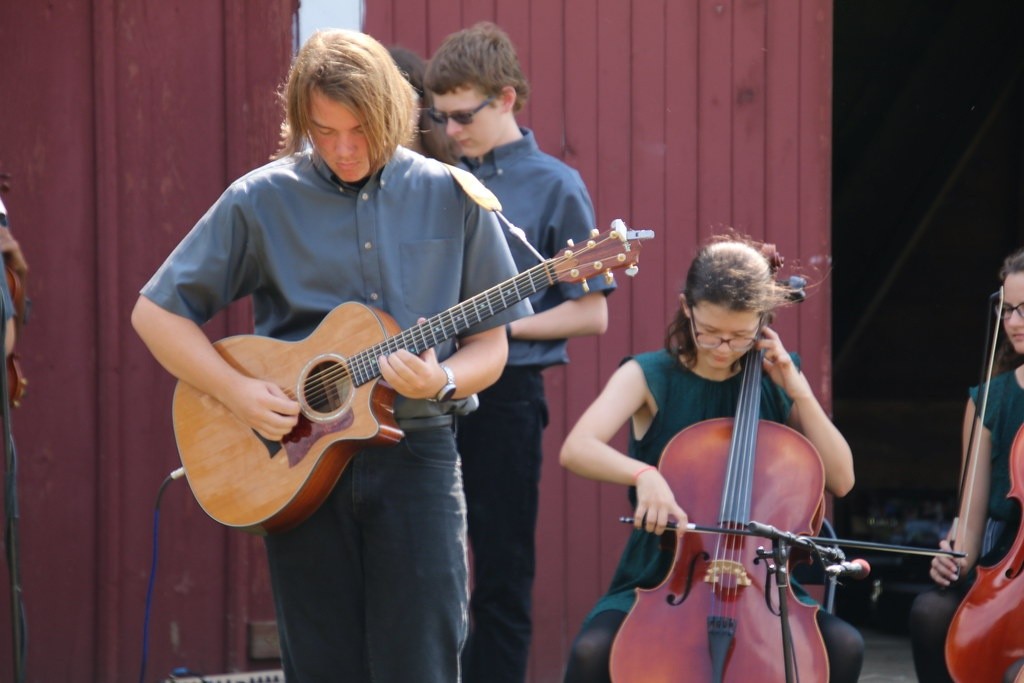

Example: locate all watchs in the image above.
[424,363,457,404]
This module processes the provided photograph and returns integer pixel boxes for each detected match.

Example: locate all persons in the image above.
[389,19,617,683]
[1,253,14,364]
[910,254,1024,683]
[557,232,868,682]
[121,28,535,683]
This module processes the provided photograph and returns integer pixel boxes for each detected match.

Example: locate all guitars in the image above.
[170,219,657,537]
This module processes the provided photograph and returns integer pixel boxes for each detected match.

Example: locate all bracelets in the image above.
[632,466,658,483]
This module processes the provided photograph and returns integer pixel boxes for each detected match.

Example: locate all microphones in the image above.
[825,559,870,580]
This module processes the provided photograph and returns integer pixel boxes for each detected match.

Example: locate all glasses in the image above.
[995,302,1024,322]
[427,94,498,125]
[687,303,764,353]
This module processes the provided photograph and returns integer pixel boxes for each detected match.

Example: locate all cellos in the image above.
[945,421,1024,683]
[608,242,832,683]
